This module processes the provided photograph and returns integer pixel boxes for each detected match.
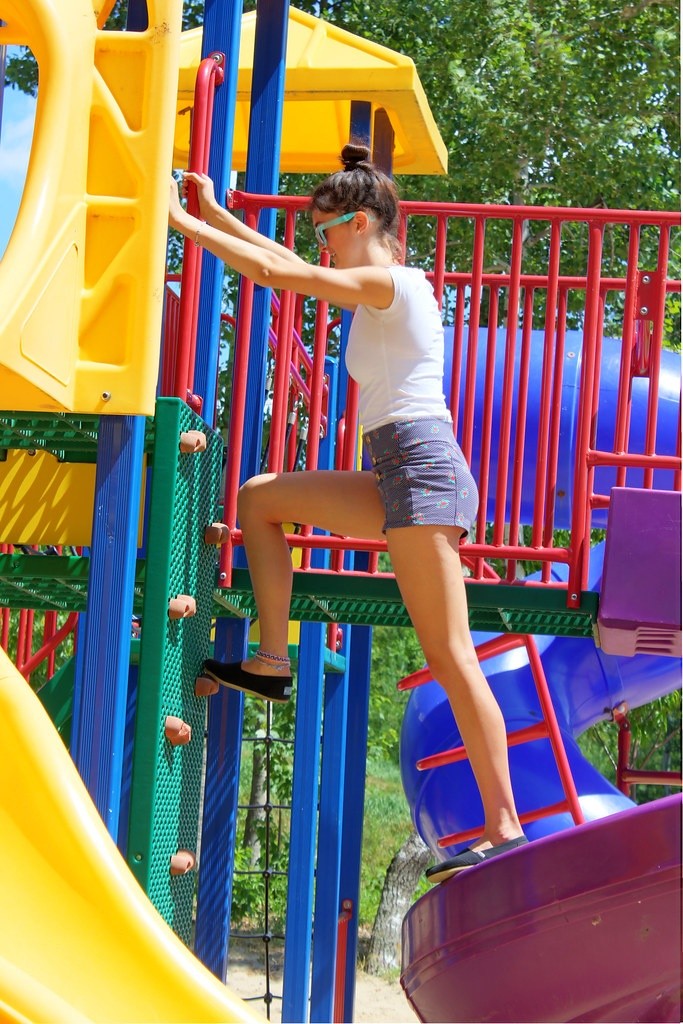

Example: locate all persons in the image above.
[167,144,534,881]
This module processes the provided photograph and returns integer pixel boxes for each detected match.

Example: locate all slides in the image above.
[0,644,267,1024]
[400,325,683,863]
[400,485,683,1024]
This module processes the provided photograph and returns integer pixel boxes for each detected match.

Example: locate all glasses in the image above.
[314,211,376,247]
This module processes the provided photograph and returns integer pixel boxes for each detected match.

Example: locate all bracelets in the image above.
[195,222,206,247]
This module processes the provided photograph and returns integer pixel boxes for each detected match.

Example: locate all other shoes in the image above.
[203,659,293,703]
[425,834,530,884]
